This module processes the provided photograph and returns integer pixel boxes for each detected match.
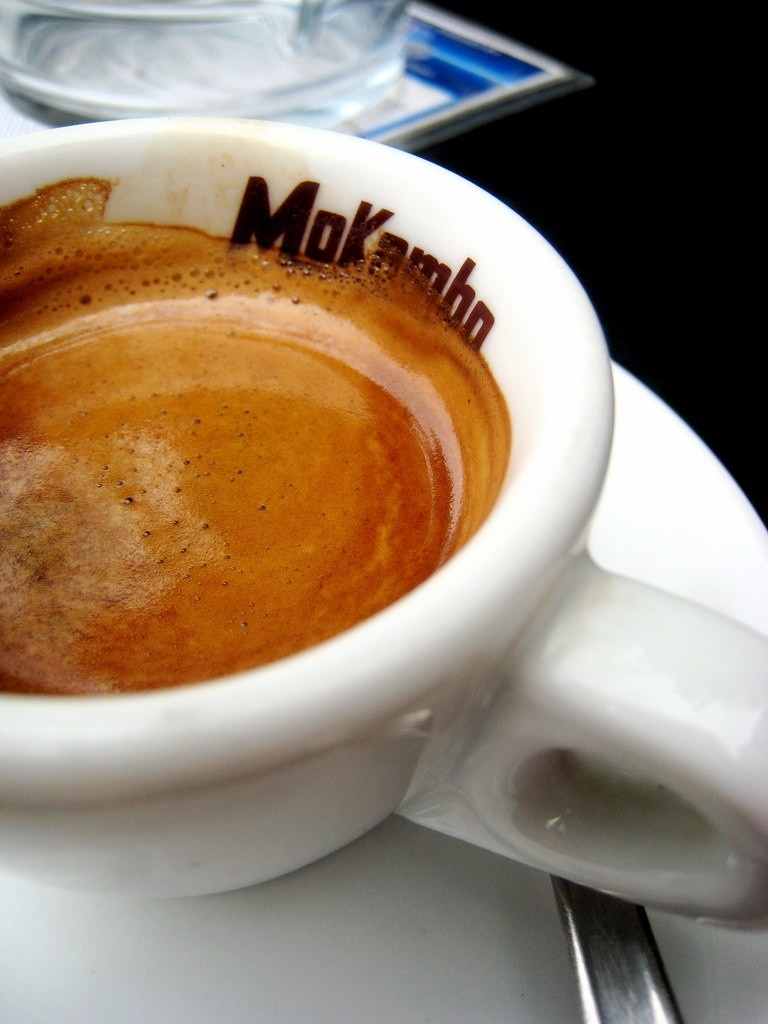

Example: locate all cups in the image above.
[0,0,413,144]
[0,119,768,942]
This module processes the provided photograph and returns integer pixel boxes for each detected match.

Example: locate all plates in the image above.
[0,361,767,1024]
[0,0,595,154]
